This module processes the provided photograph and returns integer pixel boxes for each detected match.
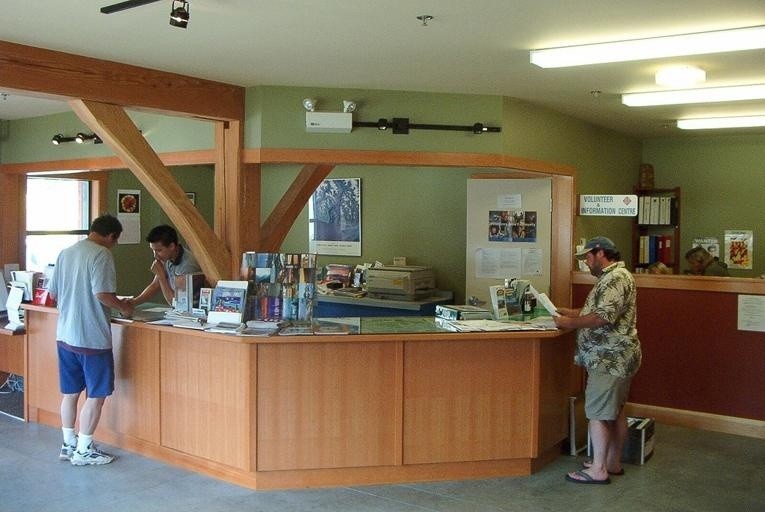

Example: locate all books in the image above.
[2,261,46,303]
[146,250,360,336]
[637,195,675,275]
[434,274,560,332]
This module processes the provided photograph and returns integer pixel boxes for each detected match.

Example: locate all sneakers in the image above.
[60,443,77,460]
[70,441,114,465]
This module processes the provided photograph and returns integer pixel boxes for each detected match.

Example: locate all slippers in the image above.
[565,469,611,485]
[583,460,624,476]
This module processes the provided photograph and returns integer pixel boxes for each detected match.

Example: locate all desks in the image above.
[0,326,27,422]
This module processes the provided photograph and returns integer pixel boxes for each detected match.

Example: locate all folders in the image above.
[638,196,671,224]
[639,236,672,265]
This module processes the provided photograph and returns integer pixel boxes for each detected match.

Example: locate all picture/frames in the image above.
[724,229,755,270]
[185,191,196,207]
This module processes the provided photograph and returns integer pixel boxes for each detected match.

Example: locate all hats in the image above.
[575,237,617,260]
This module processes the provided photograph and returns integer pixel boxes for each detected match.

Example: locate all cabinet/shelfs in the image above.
[313,292,452,317]
[631,186,679,276]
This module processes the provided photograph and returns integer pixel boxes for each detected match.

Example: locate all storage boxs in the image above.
[619,415,654,466]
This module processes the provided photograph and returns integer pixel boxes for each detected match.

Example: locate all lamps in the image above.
[677,110,764,130]
[529,26,765,71]
[473,122,483,134]
[621,85,764,110]
[49,132,101,146]
[377,119,388,131]
[168,0,190,29]
[301,97,356,133]
[657,67,707,89]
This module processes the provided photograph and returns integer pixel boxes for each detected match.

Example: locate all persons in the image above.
[45,214,134,466]
[121,224,201,308]
[684,246,730,276]
[551,236,643,486]
